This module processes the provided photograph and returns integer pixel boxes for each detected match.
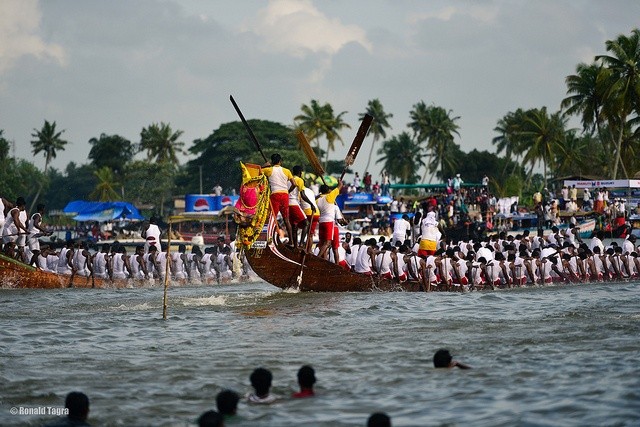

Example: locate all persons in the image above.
[198,411,224,427]
[64,391,90,425]
[286,166,315,249]
[1,154,246,296]
[341,170,640,290]
[217,390,239,415]
[250,367,272,399]
[259,153,294,247]
[432,349,452,367]
[326,200,348,265]
[298,365,316,395]
[297,184,320,253]
[316,177,343,261]
[367,412,390,426]
[279,226,289,244]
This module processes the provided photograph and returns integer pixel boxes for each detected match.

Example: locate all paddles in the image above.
[341,113,375,179]
[230,95,271,165]
[298,130,330,192]
[282,210,315,292]
[32,232,52,238]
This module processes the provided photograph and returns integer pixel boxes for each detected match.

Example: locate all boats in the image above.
[0,238,243,289]
[96,238,193,252]
[238,163,640,292]
[487,218,596,239]
